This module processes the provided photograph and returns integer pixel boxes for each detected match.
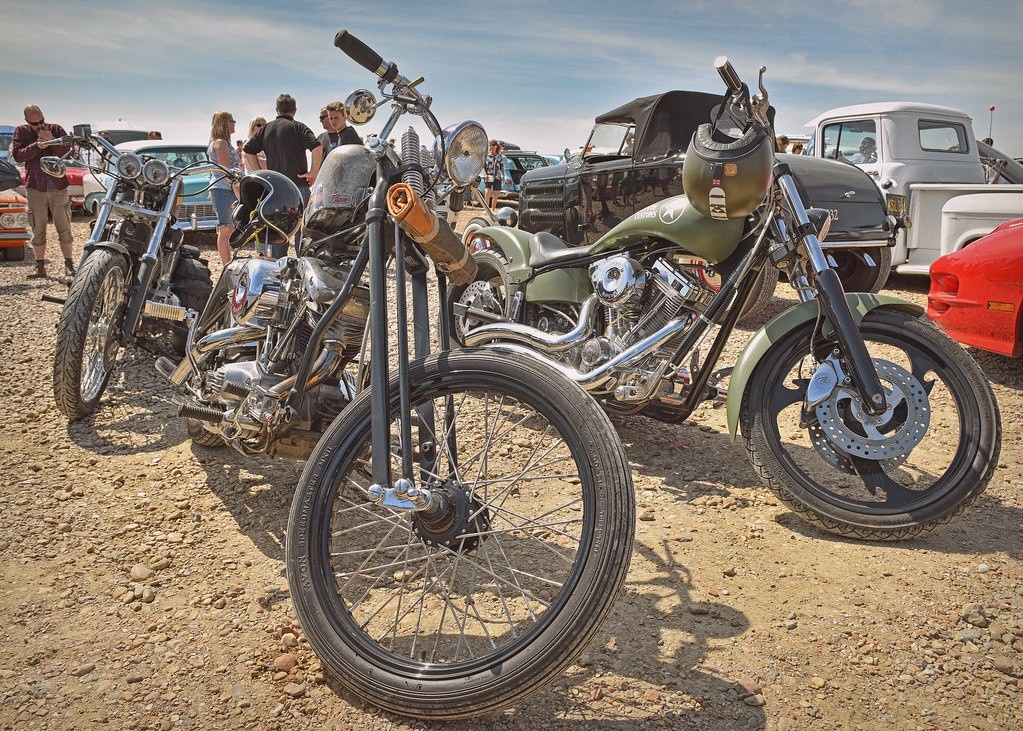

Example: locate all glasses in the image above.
[320,114,329,120]
[229,120,235,124]
[26,117,44,126]
[254,123,265,127]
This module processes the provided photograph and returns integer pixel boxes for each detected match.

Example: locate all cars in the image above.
[796,99,1022,276]
[926,220,1023,362]
[519,90,897,294]
[1,127,548,262]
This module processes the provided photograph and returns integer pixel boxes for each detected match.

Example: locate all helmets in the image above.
[232,170,305,245]
[682,122,772,220]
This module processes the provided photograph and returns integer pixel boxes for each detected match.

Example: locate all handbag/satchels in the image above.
[486,174,494,182]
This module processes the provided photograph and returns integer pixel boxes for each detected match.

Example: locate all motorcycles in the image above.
[439,55,1002,542]
[39,123,245,422]
[145,30,638,719]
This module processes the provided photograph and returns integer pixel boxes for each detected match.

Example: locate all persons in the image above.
[581,167,673,230]
[206,111,240,266]
[483,139,505,211]
[243,116,268,256]
[243,94,323,259]
[792,142,803,154]
[853,137,877,163]
[777,135,789,153]
[148,131,169,158]
[102,130,114,146]
[317,102,364,159]
[12,104,76,278]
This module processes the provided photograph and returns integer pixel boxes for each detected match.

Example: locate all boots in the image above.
[65,262,77,276]
[27,265,47,279]
